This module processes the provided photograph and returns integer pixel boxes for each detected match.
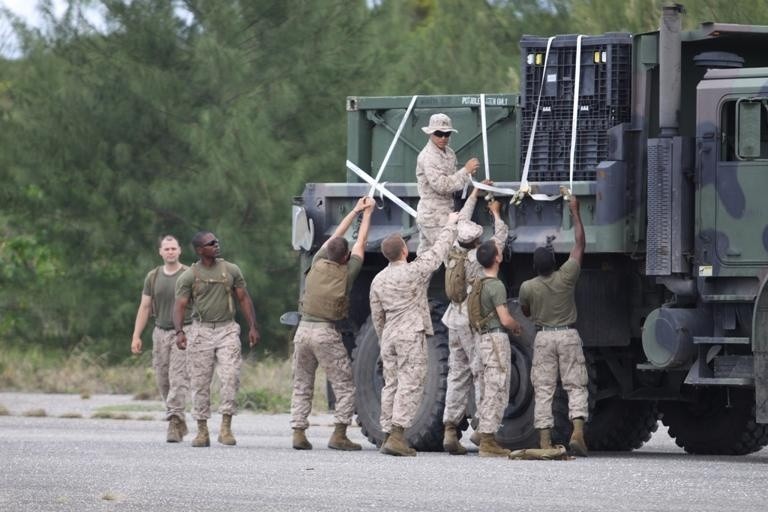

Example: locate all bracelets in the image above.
[351,209,360,216]
[175,329,185,336]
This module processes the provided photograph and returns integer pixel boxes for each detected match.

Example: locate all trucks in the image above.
[279,2,768,454]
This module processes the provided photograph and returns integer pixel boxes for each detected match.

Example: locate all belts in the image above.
[154,321,192,330]
[192,319,235,330]
[536,324,577,332]
[482,327,507,334]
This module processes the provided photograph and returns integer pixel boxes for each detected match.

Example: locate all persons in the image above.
[131,234,193,444]
[290,195,377,451]
[442,180,509,456]
[173,230,260,448]
[518,195,588,458]
[368,212,460,458]
[414,113,480,269]
[469,238,521,458]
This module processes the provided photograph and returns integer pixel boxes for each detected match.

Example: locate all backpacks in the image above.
[467,276,503,330]
[508,443,569,460]
[444,246,479,304]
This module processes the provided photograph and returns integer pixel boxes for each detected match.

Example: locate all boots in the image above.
[167,416,188,442]
[217,414,236,445]
[478,432,512,455]
[538,427,554,449]
[292,428,312,449]
[443,425,467,455]
[384,424,417,455]
[327,423,361,451]
[569,419,588,457]
[469,424,480,446]
[192,420,210,447]
[379,432,401,455]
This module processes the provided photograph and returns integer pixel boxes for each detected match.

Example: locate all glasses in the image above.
[200,238,218,249]
[433,131,452,137]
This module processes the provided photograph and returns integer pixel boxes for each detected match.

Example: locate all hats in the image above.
[421,113,459,135]
[456,220,484,244]
